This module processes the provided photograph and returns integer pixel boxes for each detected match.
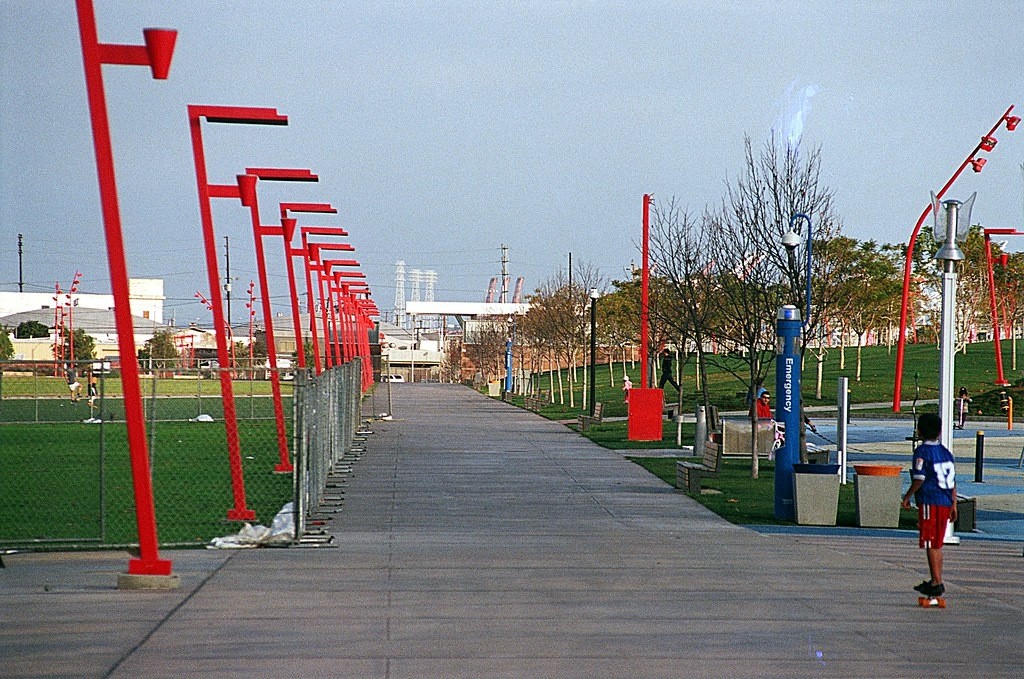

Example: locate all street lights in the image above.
[774,304,802,464]
[504,338,514,393]
[929,189,977,457]
[770,213,813,465]
[984,228,1024,385]
[52,280,62,377]
[57,305,69,377]
[73,0,181,593]
[245,278,258,379]
[65,270,83,369]
[589,286,598,416]
[892,104,1022,415]
[186,104,385,523]
[194,289,238,379]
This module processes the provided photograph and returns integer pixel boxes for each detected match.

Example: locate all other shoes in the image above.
[86,402,98,409]
[78,393,82,397]
[70,400,75,405]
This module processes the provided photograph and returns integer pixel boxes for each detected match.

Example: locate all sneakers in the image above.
[915,581,945,596]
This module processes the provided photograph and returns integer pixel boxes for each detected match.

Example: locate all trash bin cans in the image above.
[721,417,775,455]
[791,463,842,526]
[854,464,903,527]
[487,380,501,397]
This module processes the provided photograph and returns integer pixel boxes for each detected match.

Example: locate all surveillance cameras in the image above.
[781,232,800,251]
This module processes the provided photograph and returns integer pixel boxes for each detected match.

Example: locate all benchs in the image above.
[807,442,830,463]
[956,494,977,533]
[578,402,603,433]
[677,442,723,496]
[504,385,551,411]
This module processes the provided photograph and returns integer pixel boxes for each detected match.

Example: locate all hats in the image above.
[958,387,968,394]
[623,376,629,381]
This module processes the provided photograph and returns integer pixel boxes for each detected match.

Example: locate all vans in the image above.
[381,374,404,382]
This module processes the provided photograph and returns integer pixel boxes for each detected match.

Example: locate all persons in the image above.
[745,377,767,406]
[622,376,632,404]
[65,363,84,405]
[748,390,774,418]
[85,369,98,408]
[659,348,684,394]
[804,415,817,432]
[957,387,972,426]
[900,413,956,594]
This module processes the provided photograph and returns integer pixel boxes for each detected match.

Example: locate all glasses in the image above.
[762,396,770,399]
